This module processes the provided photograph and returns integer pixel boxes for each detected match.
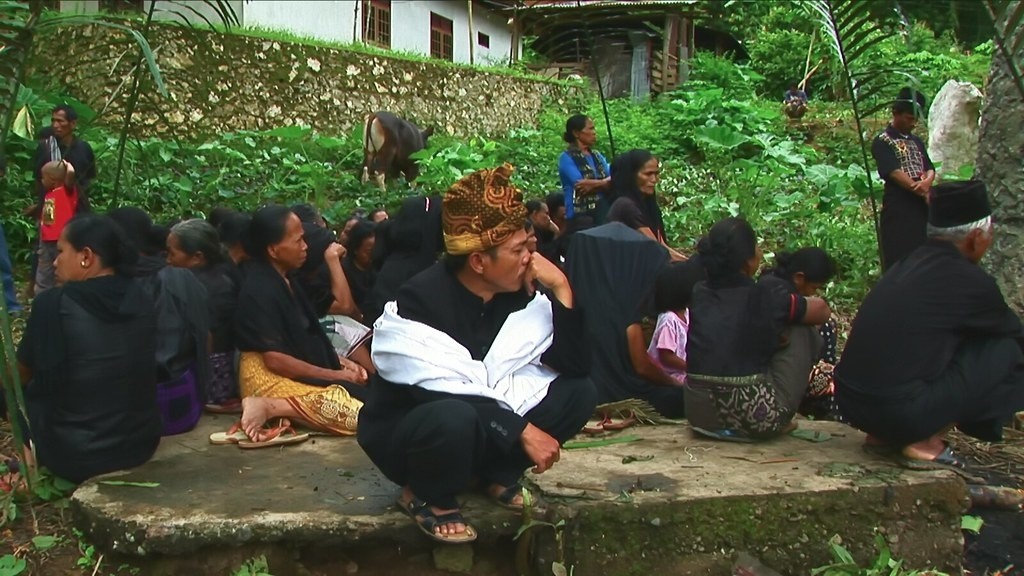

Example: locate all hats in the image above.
[440,161,532,256]
[893,87,925,115]
[925,181,995,229]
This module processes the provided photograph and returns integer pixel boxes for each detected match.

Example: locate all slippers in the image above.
[208,418,310,450]
[898,441,993,484]
[582,406,640,433]
[489,479,534,508]
[393,494,480,543]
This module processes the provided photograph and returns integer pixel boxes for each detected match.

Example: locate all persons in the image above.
[0,105,98,316]
[558,114,612,236]
[356,183,596,542]
[20,149,848,506]
[831,182,1023,483]
[870,86,935,273]
[782,82,810,123]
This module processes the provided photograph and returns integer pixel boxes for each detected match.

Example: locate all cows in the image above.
[357,109,435,195]
